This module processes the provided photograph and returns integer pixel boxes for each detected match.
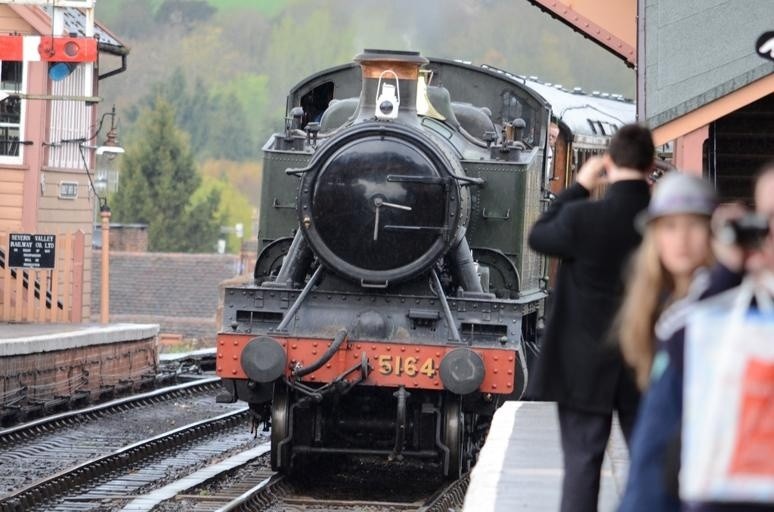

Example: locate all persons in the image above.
[622,168,719,393]
[622,172,774,510]
[527,124,655,512]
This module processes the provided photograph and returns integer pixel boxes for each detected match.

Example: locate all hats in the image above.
[632,172,718,236]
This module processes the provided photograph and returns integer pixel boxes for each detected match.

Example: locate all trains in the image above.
[215,48,673,480]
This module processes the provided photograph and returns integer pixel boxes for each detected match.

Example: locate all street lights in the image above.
[79,105,126,324]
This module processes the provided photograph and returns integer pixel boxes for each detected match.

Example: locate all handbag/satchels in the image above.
[678,273,774,502]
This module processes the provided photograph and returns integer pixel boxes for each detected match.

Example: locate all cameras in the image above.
[716,216,770,248]
